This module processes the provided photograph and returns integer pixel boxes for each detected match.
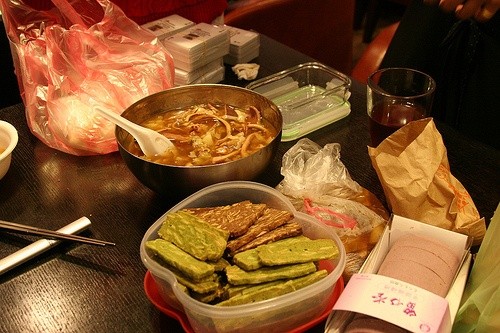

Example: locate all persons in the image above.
[378,0,500,147]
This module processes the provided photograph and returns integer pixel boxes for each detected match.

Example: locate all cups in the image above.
[365,66,437,148]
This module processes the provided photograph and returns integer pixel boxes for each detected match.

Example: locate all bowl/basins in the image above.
[138,180,347,332]
[243,59,352,131]
[115,83,284,201]
[0,119,19,181]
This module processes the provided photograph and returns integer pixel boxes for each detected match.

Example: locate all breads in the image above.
[343,234,459,333]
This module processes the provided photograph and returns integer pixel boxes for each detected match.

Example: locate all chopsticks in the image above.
[0,219,117,247]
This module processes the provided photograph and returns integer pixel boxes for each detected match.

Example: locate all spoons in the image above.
[92,104,172,156]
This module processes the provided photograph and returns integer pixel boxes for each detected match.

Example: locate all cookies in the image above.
[147,201,339,333]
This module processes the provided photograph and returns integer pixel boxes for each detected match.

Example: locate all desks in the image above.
[0,25,500,333]
